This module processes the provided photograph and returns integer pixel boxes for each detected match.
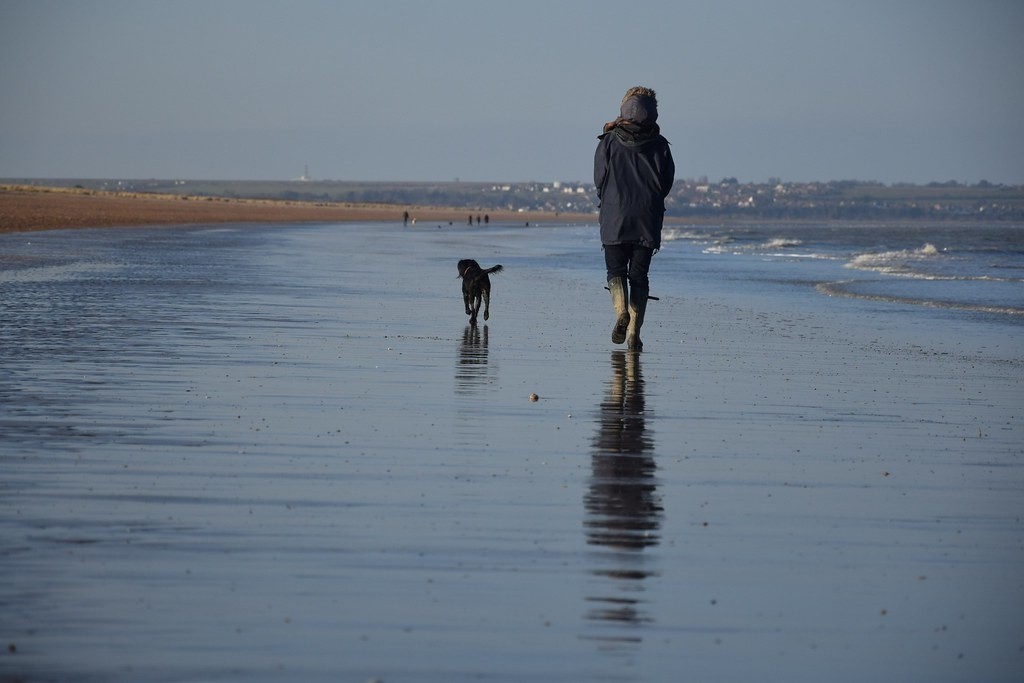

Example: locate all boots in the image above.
[628,301,647,352]
[608,276,631,344]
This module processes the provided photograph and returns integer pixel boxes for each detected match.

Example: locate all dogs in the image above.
[456,259,503,325]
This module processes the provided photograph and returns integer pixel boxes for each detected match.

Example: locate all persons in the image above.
[466,213,489,227]
[402,210,410,226]
[592,86,676,350]
